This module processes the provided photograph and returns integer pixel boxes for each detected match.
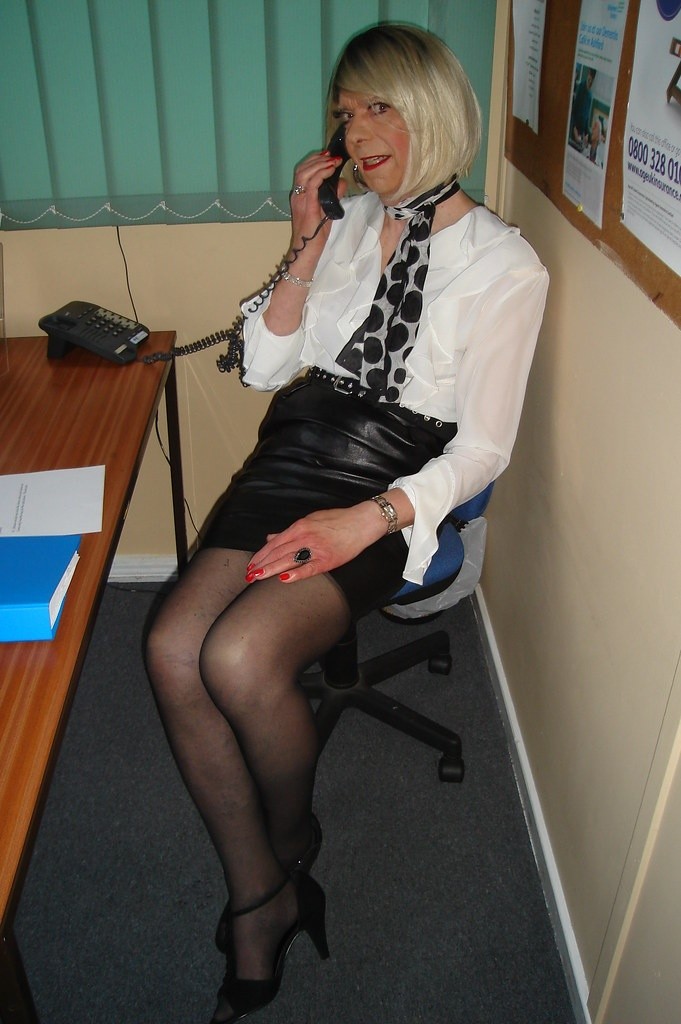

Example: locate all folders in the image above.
[1,535,85,644]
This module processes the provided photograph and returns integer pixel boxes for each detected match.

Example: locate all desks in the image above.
[0,330,190,1024]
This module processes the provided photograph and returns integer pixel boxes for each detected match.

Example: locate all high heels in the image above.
[211,814,330,1024]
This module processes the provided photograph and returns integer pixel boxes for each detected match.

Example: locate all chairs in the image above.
[296,482,493,784]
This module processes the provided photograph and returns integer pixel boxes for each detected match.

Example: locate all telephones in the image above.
[317,120,350,220]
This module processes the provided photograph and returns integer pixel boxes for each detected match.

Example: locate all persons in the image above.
[144,24,550,1024]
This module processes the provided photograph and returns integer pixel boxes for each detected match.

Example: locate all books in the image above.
[0,534,83,642]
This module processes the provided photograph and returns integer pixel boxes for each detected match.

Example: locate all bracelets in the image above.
[281,265,314,288]
[371,496,398,535]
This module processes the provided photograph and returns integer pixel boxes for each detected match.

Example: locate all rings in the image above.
[293,184,304,195]
[295,548,311,562]
